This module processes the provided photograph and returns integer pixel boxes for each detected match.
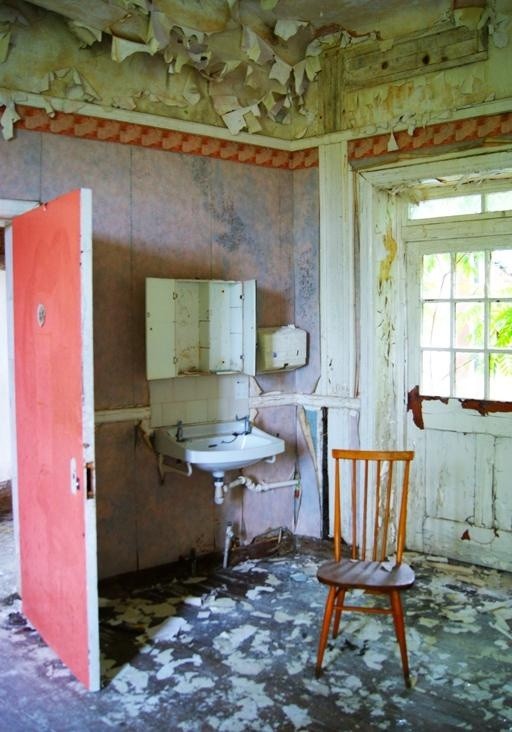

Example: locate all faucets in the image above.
[234,413,250,434]
[175,420,184,442]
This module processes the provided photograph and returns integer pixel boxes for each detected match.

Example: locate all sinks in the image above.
[151,420,284,477]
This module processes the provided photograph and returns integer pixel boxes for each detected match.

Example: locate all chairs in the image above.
[317,450,416,690]
